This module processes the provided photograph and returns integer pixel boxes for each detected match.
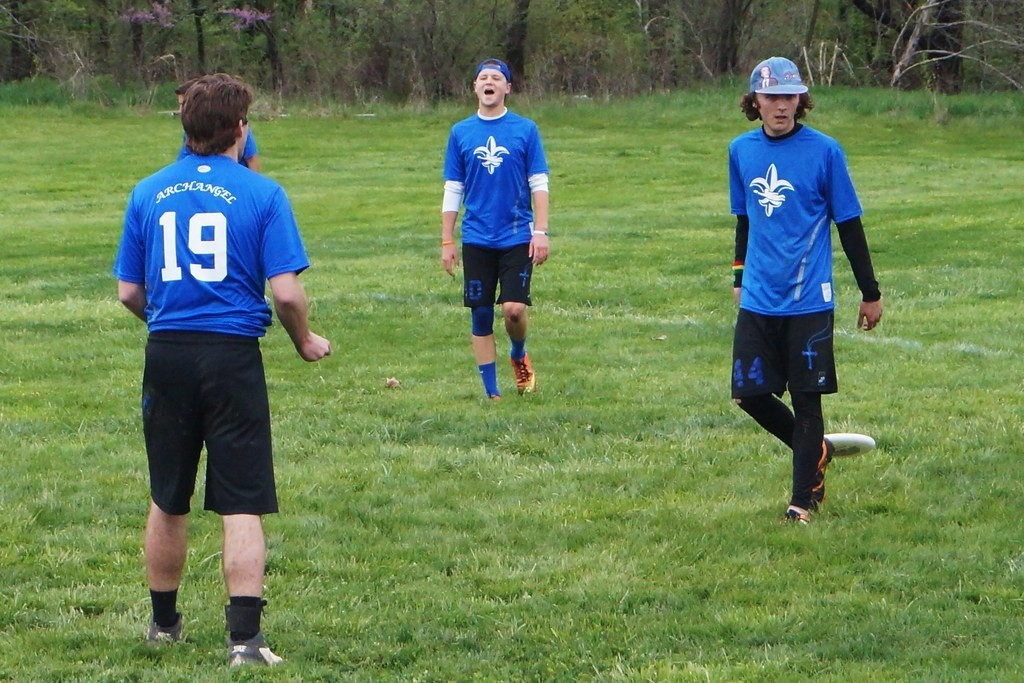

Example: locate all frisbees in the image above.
[821,432,876,456]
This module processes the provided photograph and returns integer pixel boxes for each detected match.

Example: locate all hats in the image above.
[475,57,511,82]
[749,56,808,95]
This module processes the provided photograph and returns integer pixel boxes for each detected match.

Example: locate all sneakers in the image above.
[510,349,536,396]
[227,628,283,670]
[488,393,502,401]
[148,611,183,648]
[809,437,835,512]
[784,504,811,528]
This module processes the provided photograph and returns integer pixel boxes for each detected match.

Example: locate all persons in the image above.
[441,59,549,398]
[118,72,329,670]
[728,57,882,524]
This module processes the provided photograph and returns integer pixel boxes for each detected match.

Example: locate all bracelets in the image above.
[533,230,548,235]
[441,241,454,245]
